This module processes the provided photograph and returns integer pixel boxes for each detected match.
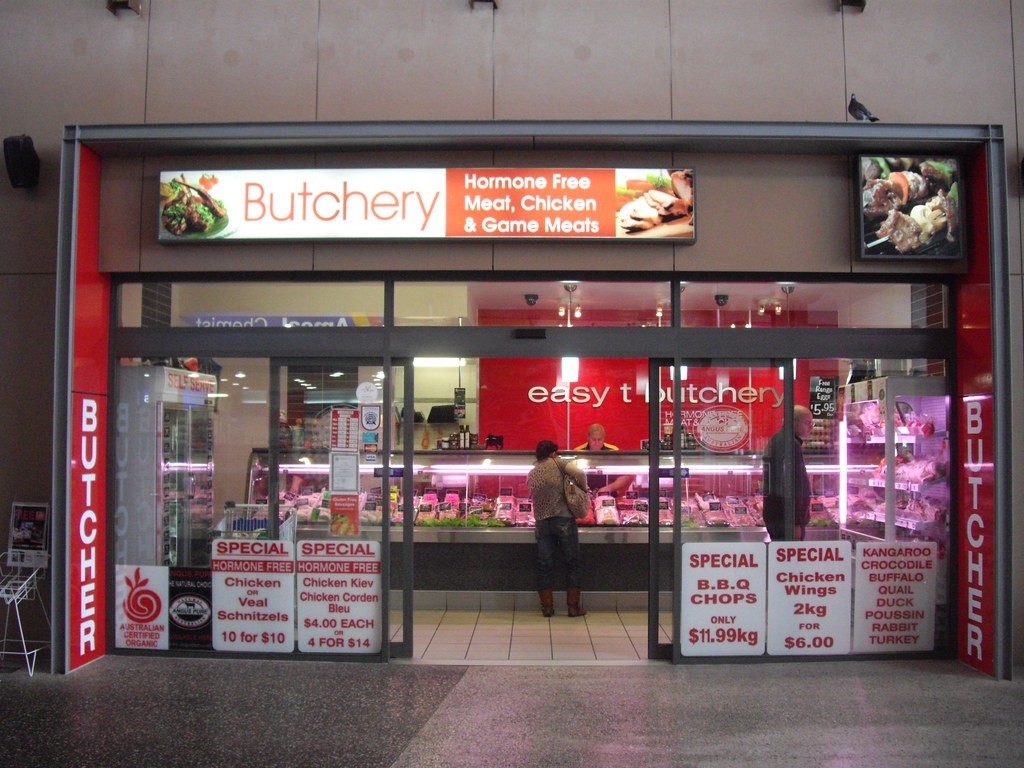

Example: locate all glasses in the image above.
[800,419,815,426]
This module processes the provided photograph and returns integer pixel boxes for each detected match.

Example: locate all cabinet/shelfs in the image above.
[118,365,218,569]
[838,377,950,609]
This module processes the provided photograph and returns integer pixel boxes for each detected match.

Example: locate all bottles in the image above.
[681,426,684,449]
[436,425,478,451]
[661,435,673,450]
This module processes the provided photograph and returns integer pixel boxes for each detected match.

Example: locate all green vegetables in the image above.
[164,181,224,226]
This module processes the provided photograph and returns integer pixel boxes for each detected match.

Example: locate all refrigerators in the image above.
[115,365,218,566]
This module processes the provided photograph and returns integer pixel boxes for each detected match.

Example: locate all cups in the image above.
[663,424,673,435]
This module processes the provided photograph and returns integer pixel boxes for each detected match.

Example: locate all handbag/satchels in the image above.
[552,457,589,518]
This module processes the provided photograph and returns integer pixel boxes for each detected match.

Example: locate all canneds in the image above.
[687,433,696,450]
[664,434,673,450]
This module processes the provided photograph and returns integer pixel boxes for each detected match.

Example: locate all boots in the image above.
[567,587,587,617]
[539,588,554,617]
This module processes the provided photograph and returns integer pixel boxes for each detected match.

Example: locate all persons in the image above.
[526,440,591,617]
[763,405,816,541]
[574,424,630,499]
